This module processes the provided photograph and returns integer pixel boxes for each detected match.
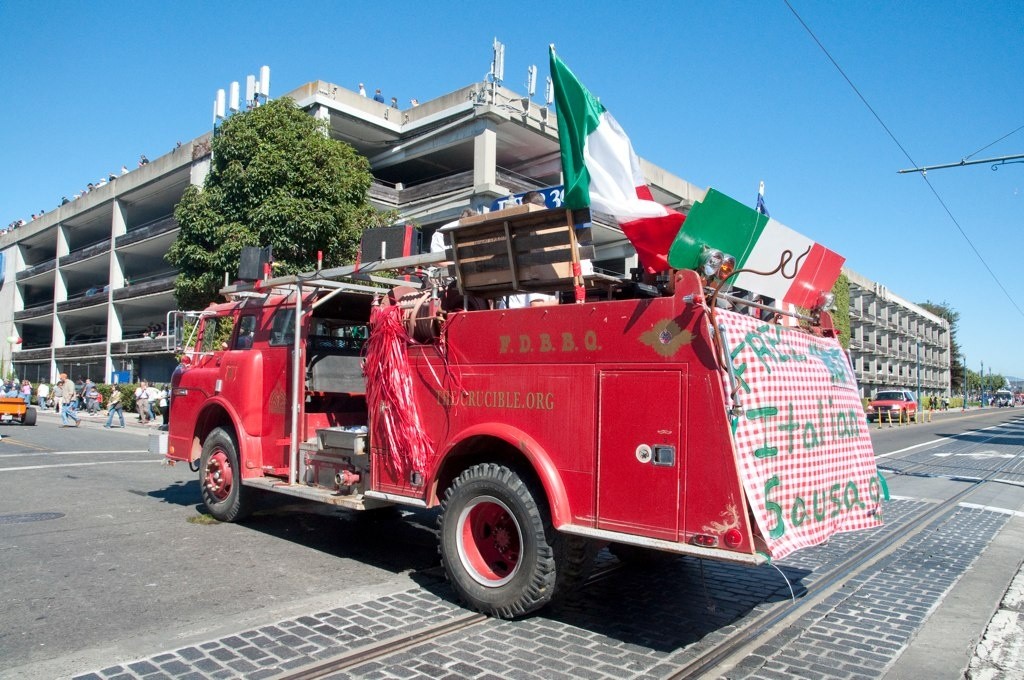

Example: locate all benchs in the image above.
[307,340,369,393]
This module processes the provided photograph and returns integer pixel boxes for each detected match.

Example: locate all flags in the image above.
[549,44,688,276]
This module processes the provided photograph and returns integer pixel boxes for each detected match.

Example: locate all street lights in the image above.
[915,337,922,413]
[988,365,993,401]
[980,359,986,408]
[962,352,969,409]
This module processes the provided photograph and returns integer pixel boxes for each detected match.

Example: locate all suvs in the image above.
[866,389,918,423]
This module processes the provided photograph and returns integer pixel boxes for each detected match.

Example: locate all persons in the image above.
[430,208,506,310]
[497,190,562,310]
[866,399,874,412]
[1,139,182,241]
[391,97,399,109]
[373,88,384,104]
[142,322,168,339]
[929,394,951,413]
[411,99,420,108]
[0,372,172,442]
[253,97,261,107]
[246,104,253,112]
[358,82,366,97]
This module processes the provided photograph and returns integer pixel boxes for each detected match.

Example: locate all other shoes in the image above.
[59,425,68,428]
[76,419,81,426]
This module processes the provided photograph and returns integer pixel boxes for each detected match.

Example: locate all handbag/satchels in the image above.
[114,401,122,410]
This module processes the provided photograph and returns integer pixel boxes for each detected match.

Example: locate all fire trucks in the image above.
[166,194,886,622]
